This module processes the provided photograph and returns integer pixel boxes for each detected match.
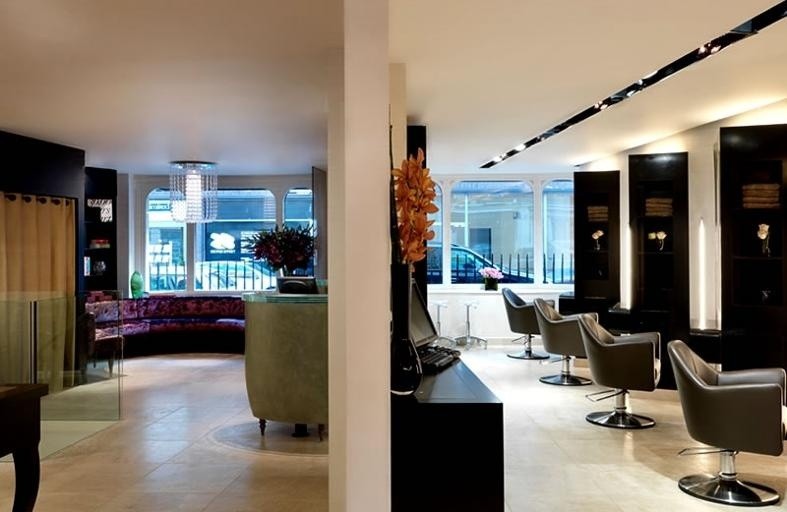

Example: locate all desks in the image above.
[240,293,328,441]
[0,382,49,512]
[390,350,503,512]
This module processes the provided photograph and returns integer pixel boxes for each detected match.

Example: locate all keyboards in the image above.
[419,345,461,371]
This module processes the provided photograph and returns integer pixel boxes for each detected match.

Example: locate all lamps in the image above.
[166,160,219,225]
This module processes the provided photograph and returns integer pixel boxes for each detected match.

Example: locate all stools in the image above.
[454,300,488,348]
[428,299,453,344]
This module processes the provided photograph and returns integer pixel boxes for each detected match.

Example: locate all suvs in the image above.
[426,240,553,286]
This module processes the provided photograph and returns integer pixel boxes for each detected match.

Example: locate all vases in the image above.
[762,238,769,252]
[657,240,664,250]
[597,241,601,250]
[484,278,499,292]
[130,269,145,299]
[391,264,422,398]
[287,272,294,276]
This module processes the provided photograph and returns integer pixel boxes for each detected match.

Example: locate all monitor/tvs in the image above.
[407,277,439,349]
[310,165,327,281]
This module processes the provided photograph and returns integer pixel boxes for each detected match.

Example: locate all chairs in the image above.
[576,313,664,430]
[500,288,555,362]
[665,338,787,508]
[531,297,600,387]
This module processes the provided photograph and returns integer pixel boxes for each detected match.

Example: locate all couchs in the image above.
[84,292,247,363]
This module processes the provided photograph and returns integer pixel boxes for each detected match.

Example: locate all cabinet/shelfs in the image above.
[582,198,611,281]
[81,218,116,292]
[730,170,786,311]
[642,183,677,287]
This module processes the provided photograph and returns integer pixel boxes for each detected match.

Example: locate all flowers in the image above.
[646,230,668,250]
[478,265,505,279]
[247,224,318,271]
[755,222,773,250]
[590,229,605,250]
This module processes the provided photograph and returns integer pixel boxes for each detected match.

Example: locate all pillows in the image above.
[275,276,320,295]
[281,281,307,294]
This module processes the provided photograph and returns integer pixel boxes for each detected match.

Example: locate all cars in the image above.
[144,256,317,294]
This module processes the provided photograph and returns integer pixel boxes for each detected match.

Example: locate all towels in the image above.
[744,185,780,209]
[589,206,608,222]
[647,198,674,216]
